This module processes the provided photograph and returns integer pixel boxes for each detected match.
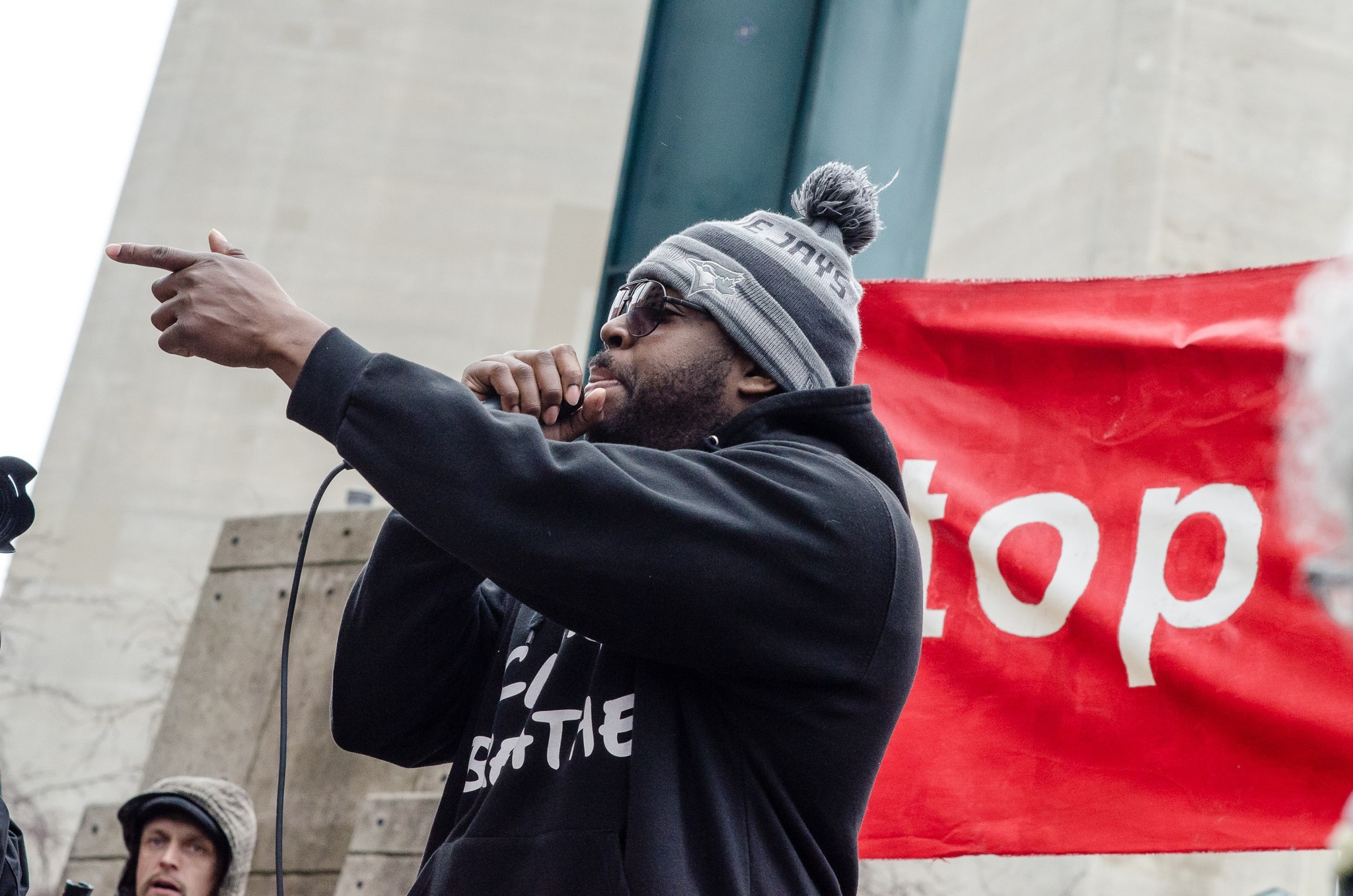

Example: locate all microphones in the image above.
[482,381,586,419]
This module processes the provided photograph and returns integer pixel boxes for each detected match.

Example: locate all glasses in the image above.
[604,277,707,337]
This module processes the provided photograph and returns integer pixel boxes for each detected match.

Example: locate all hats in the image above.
[627,161,882,391]
[129,794,224,851]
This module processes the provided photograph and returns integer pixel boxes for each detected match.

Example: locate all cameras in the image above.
[0,456,38,553]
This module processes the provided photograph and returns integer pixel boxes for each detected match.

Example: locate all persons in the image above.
[1280,250,1353,896]
[103,163,924,896]
[115,776,259,896]
